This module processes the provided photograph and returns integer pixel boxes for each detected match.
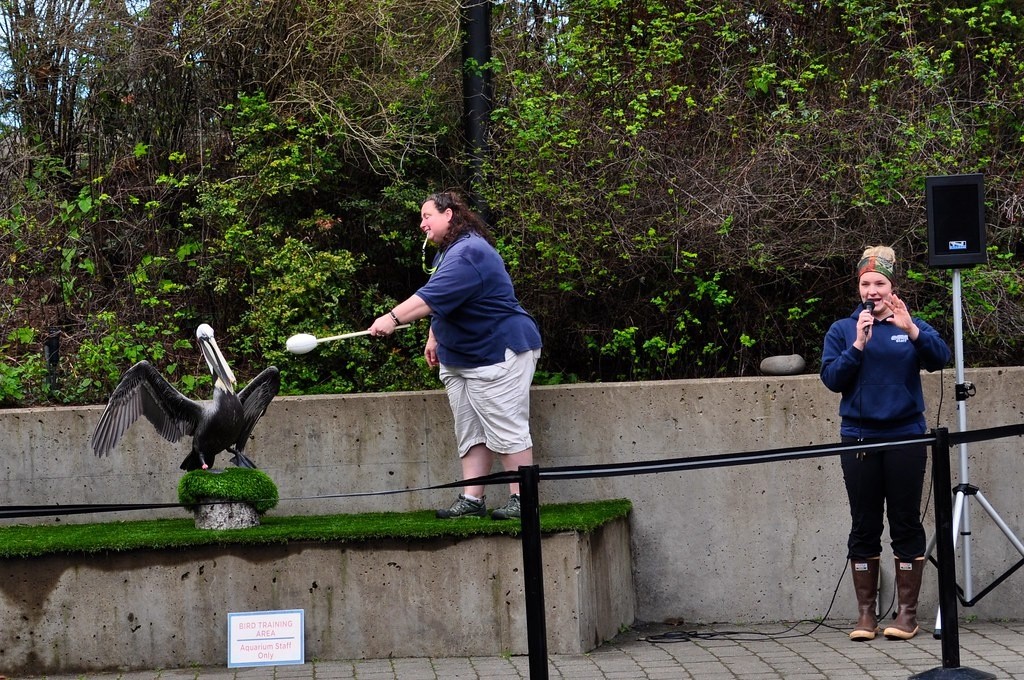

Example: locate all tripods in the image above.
[893,269,1024,639]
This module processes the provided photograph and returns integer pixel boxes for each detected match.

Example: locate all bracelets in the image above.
[388,311,402,326]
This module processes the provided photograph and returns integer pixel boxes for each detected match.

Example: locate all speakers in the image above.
[925,173,986,269]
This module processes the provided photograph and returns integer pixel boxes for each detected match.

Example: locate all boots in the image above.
[882,556,928,639]
[844,556,879,642]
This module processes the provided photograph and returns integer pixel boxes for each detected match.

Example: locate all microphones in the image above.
[863,300,875,335]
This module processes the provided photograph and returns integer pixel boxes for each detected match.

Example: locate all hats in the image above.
[856,241,895,285]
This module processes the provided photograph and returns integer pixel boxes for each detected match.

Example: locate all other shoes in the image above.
[490,493,520,519]
[435,492,488,519]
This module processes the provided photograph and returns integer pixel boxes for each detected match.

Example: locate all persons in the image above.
[367,193,540,519]
[820,246,951,641]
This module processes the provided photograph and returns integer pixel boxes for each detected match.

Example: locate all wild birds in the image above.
[92,323,279,470]
